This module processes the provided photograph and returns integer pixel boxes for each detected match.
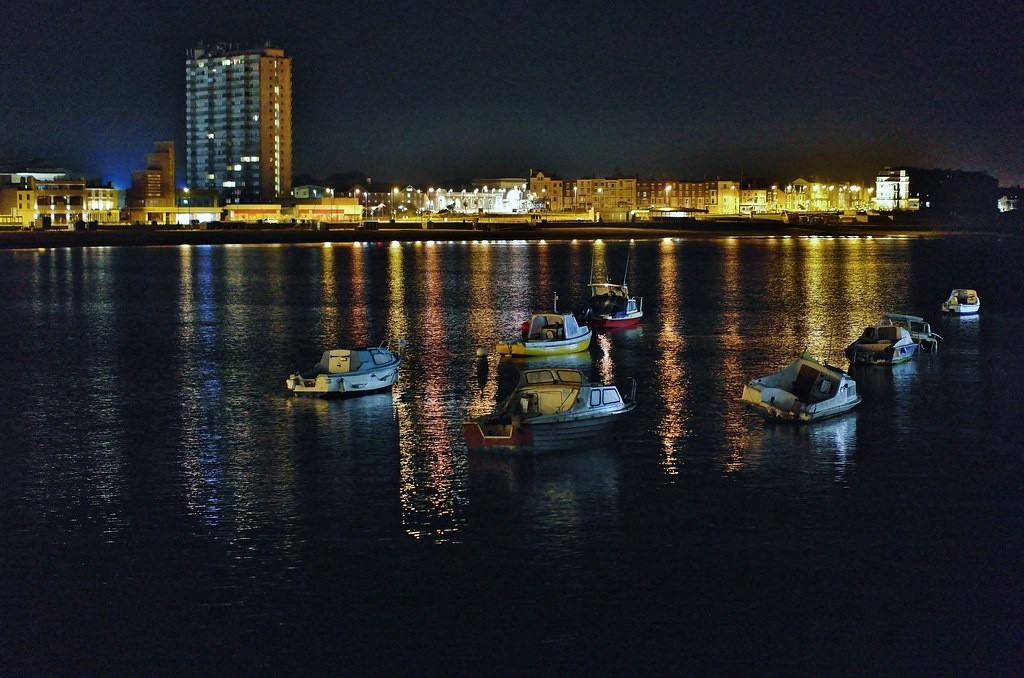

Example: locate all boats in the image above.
[740,346,862,421]
[848,313,932,365]
[461,368,640,453]
[286,340,405,392]
[577,247,644,329]
[942,289,981,314]
[495,292,592,356]
